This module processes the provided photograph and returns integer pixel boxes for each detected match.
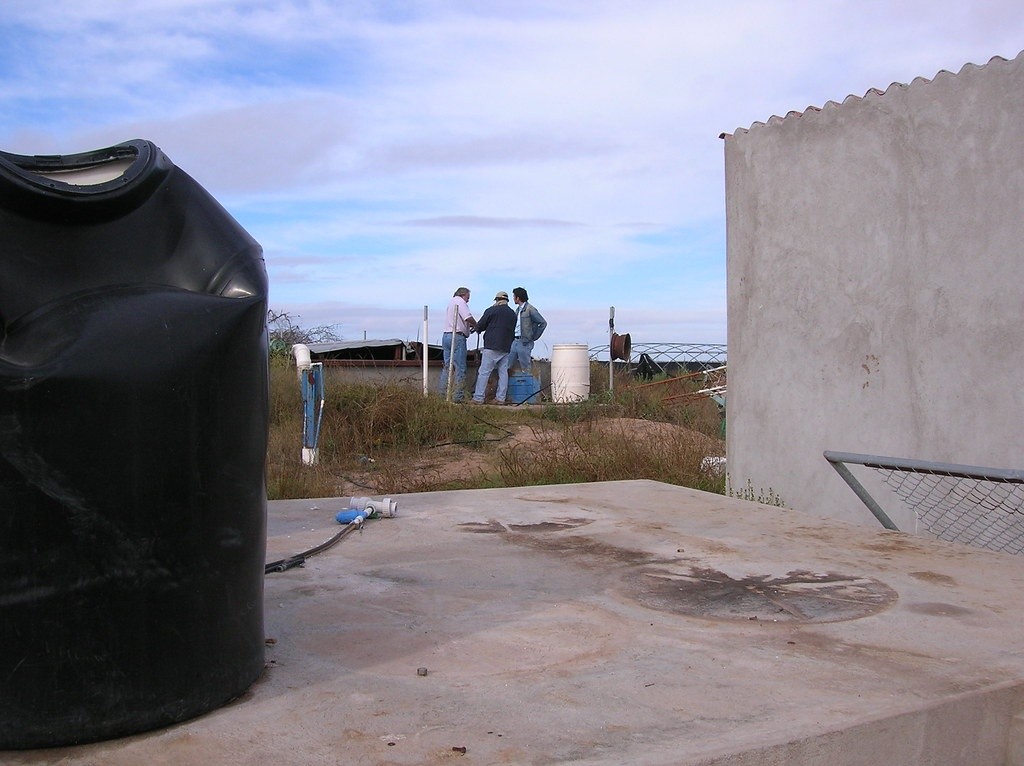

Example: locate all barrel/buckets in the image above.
[508,371,533,403]
[551,344,590,403]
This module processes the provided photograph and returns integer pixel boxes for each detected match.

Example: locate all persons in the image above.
[438,287,477,404]
[506,287,547,404]
[468,291,518,405]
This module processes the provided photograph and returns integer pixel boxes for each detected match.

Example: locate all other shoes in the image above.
[467,399,484,405]
[488,399,504,404]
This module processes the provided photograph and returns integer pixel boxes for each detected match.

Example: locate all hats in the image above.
[493,291,508,301]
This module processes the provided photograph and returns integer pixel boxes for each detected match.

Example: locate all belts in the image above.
[444,332,464,335]
[515,336,521,339]
